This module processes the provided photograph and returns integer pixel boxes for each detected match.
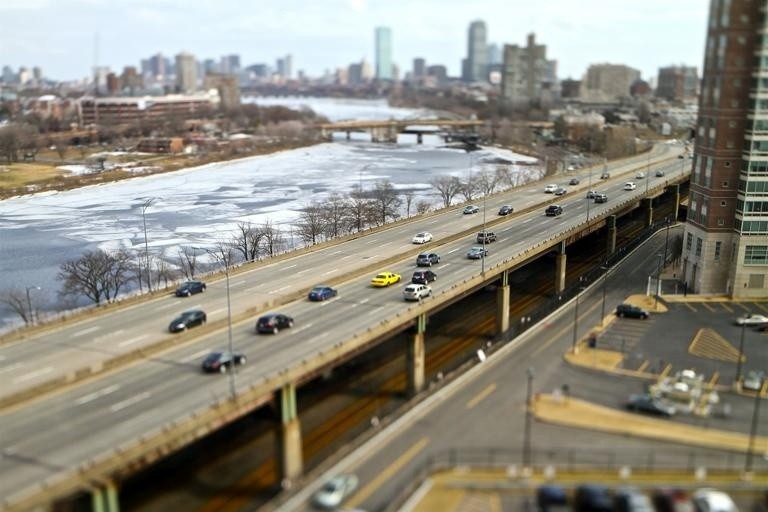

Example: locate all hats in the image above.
[309,285,337,302]
[200,349,248,373]
[370,272,402,287]
[413,269,438,284]
[458,168,666,261]
[664,138,694,160]
[49,140,107,150]
[412,231,434,245]
[176,280,207,299]
[617,303,649,321]
[404,283,432,301]
[656,487,697,512]
[169,310,206,334]
[736,312,767,326]
[693,488,739,511]
[623,366,728,427]
[311,469,358,508]
[256,313,295,336]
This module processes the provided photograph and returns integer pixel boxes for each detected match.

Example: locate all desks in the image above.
[601,266,607,329]
[523,364,537,465]
[736,311,746,381]
[191,245,238,398]
[656,254,662,308]
[747,374,768,475]
[574,285,585,353]
[25,285,41,326]
[665,217,670,265]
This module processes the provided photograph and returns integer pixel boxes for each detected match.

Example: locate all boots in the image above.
[417,252,441,267]
[534,484,573,511]
[615,485,655,512]
[575,482,613,510]
